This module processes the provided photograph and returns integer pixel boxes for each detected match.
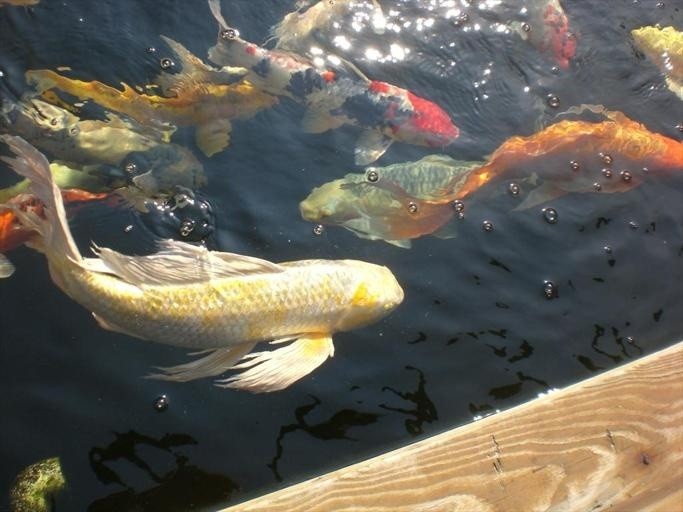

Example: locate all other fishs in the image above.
[0,0,683,259]
